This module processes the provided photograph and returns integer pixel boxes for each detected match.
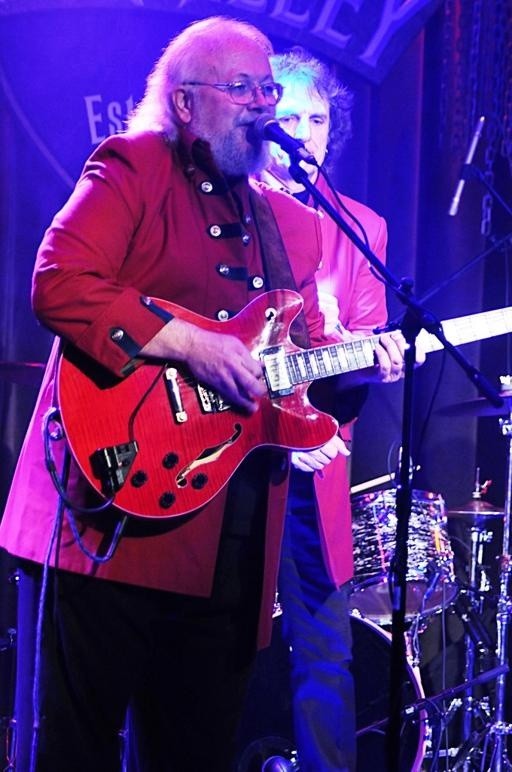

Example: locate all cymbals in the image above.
[428,393,507,429]
[444,500,504,527]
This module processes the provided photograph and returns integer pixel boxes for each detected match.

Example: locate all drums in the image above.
[352,606,431,770]
[349,485,457,624]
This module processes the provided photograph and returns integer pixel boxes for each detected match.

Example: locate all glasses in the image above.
[183,80,284,106]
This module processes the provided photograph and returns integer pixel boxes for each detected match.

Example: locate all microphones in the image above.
[251,113,316,164]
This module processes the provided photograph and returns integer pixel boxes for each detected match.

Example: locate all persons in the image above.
[2,15,426,765]
[124,51,388,766]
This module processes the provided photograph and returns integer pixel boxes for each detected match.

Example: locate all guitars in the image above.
[51,285,511,532]
